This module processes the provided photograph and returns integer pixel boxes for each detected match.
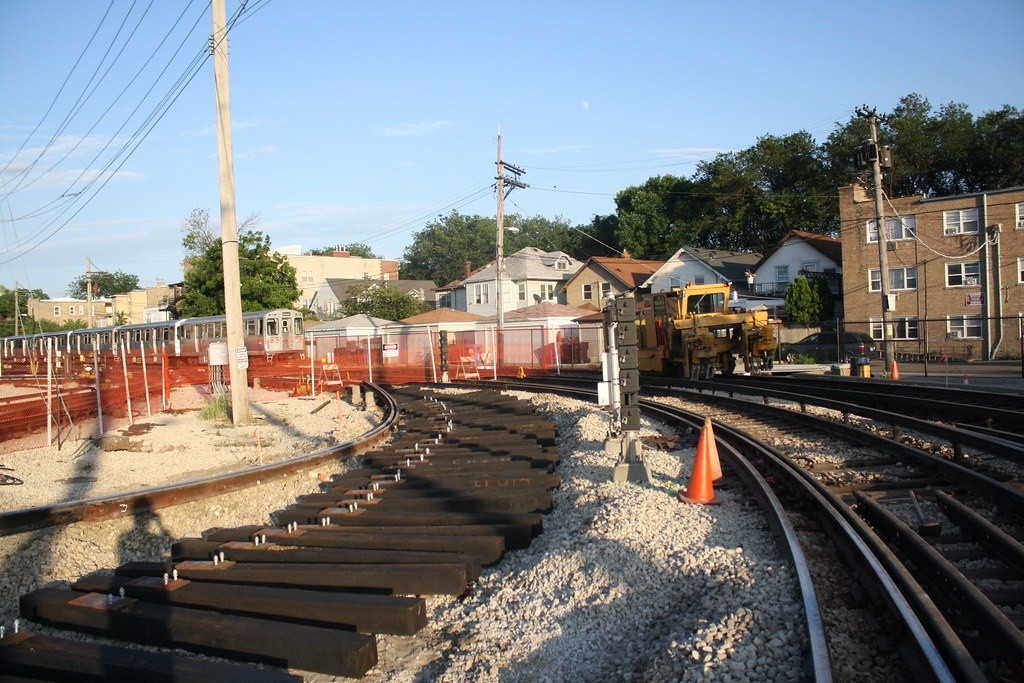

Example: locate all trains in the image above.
[1,306,306,363]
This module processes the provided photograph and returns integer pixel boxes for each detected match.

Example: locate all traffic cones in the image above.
[679,427,721,506]
[891,360,900,380]
[702,418,728,491]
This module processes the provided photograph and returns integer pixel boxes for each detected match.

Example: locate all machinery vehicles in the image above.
[637,279,778,381]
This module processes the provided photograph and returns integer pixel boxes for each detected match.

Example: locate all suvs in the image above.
[781,331,877,364]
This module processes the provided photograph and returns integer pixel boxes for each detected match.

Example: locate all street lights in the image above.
[494,227,522,369]
